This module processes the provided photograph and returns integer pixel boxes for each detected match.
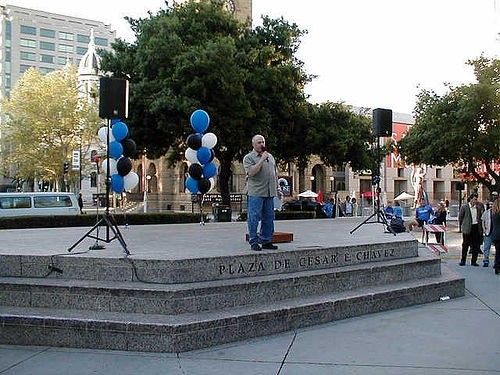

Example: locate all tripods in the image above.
[349,137,396,237]
[68,119,131,256]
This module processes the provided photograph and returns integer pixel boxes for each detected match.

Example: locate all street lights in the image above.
[147,175,151,195]
[329,176,335,193]
[309,176,316,193]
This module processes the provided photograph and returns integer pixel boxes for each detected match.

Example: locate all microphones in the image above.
[262,147,269,162]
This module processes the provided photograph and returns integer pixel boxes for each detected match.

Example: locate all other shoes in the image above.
[483,263,488,267]
[495,267,500,273]
[471,258,479,266]
[251,244,261,251]
[262,243,278,249]
[459,259,466,266]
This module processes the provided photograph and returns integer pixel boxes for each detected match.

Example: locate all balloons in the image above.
[185,111,219,195]
[97,119,139,193]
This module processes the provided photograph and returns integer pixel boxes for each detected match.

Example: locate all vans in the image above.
[0,192,82,217]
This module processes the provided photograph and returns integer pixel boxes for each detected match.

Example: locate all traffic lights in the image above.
[455,182,466,190]
[64,161,70,175]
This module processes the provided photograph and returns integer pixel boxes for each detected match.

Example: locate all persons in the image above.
[243,135,279,251]
[459,193,481,267]
[321,195,357,218]
[468,192,500,275]
[384,201,402,220]
[407,198,449,243]
[78,194,83,211]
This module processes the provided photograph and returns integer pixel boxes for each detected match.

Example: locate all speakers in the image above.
[99,77,129,119]
[373,108,392,137]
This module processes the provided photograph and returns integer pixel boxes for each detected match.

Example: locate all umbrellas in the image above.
[394,192,415,207]
[297,190,319,202]
